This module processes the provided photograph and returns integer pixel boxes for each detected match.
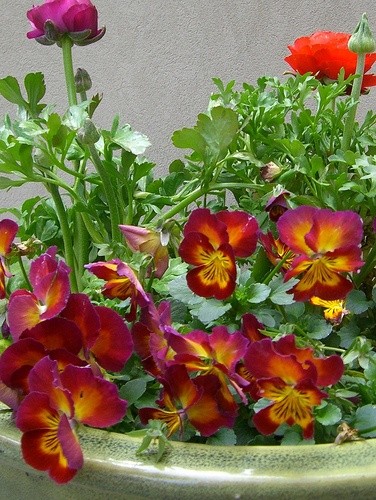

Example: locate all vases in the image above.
[1,402,375,500]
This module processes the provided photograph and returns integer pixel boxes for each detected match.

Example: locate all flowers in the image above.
[0,1,375,483]
